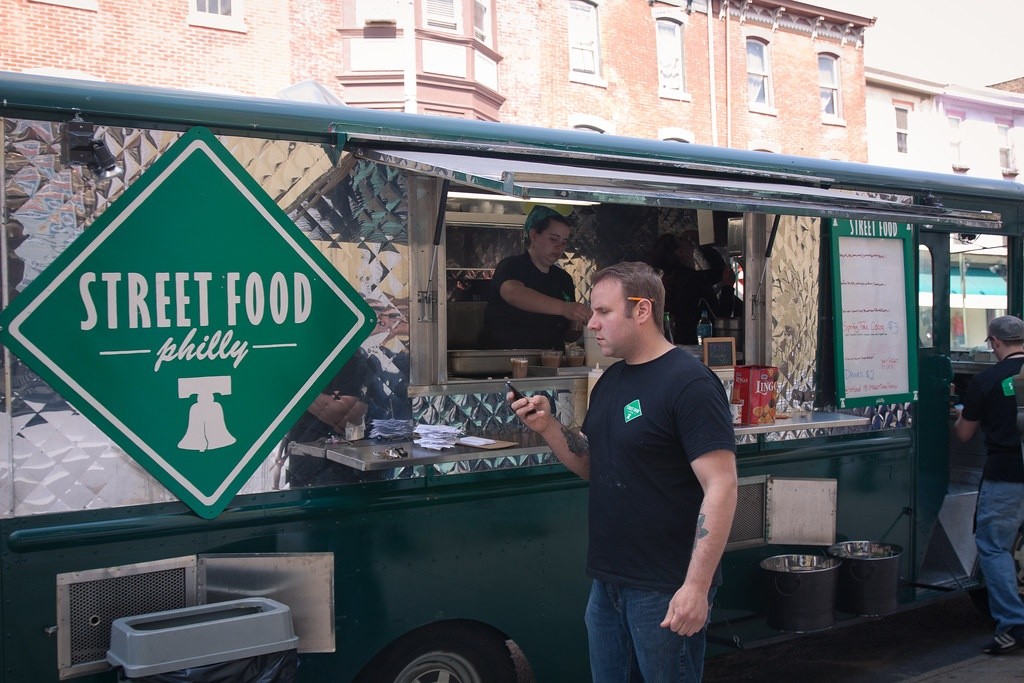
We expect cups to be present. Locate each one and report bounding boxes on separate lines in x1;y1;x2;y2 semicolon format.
510;358;528;378
540;351;564;366
566;349;585;366
568;319;583;331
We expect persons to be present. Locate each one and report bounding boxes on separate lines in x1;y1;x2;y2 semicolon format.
649;235;744;345
955;316;1024;655
475;205;592;350
286;298;409;487
505;262;739;683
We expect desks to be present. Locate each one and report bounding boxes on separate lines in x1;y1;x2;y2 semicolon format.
328;411;870;472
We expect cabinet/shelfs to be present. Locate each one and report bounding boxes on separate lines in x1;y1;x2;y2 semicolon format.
446;196;531;281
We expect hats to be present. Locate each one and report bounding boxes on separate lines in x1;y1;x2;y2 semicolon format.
984;315;1024;342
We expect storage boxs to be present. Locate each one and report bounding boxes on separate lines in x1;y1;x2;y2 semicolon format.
735;365;779;426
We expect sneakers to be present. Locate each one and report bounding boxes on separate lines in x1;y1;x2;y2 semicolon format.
980;625;1024;655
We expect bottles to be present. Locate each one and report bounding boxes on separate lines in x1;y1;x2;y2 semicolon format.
664;312;674;345
587;363;605;411
697;311;713;345
727;381;743;427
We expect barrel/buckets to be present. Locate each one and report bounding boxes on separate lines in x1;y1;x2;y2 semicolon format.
759;554;843;634
828;539;904;615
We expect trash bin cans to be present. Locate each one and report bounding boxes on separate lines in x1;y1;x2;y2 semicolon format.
97;593;307;683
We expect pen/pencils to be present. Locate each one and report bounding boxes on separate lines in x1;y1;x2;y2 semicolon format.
625;296;657;304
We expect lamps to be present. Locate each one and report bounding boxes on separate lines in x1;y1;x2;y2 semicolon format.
88;139;124;179
448;192;601;206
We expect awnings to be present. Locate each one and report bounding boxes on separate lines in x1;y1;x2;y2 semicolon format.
353;141;1002;292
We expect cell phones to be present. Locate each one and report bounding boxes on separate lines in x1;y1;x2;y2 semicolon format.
506;381;536;415
